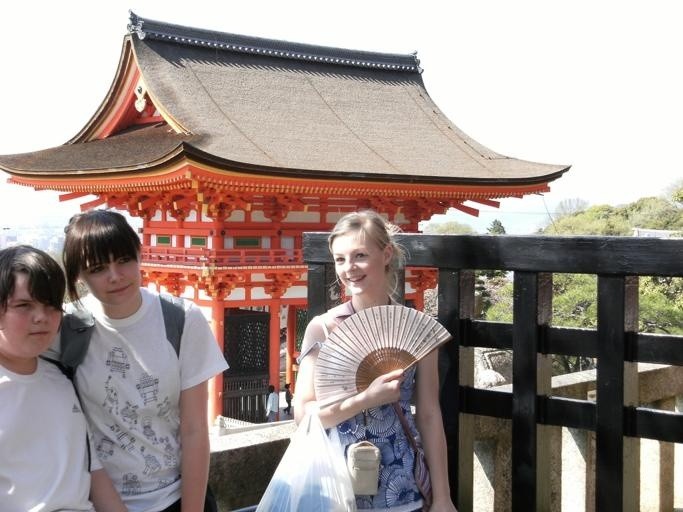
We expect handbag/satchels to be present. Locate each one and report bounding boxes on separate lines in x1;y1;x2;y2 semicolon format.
346;439;381;496
411;446;432;508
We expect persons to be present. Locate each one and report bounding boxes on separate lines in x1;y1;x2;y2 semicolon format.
0;244;127;512
283;383;293;416
264;385;279;423
294;208;458;512
46;210;232;512
